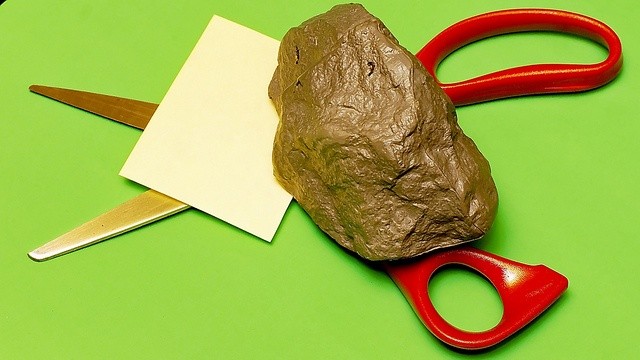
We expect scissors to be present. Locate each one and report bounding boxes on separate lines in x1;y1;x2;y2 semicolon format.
28;9;623;351
